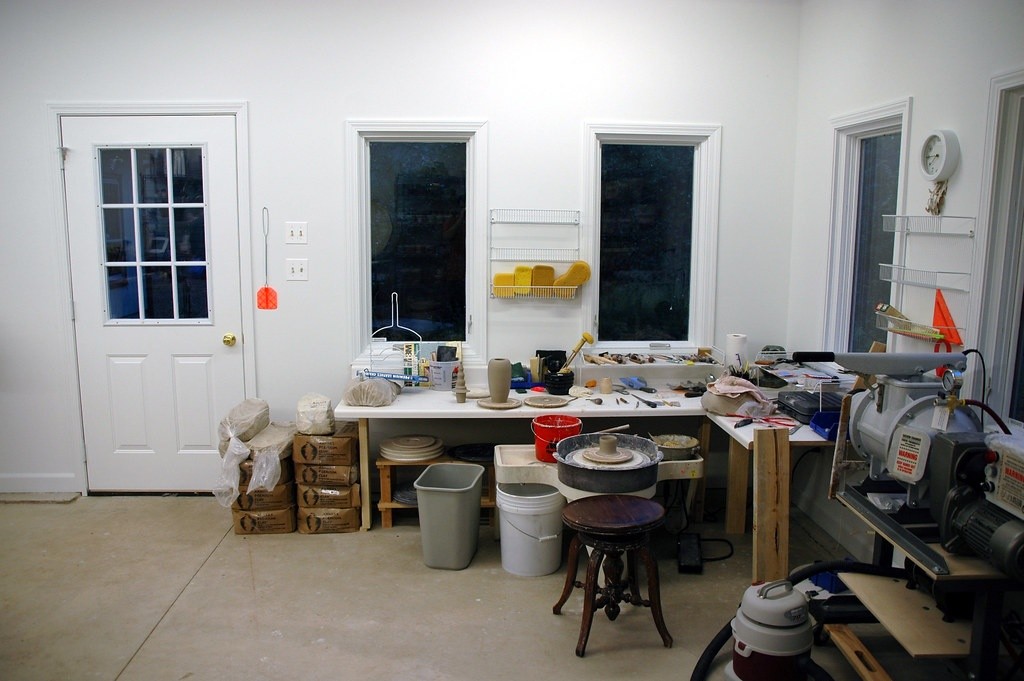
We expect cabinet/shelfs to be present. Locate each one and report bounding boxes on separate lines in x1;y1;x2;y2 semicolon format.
375;445;496;528
490;209;579;299
876;214;977;347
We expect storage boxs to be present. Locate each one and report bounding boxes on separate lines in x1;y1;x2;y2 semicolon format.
876;303;911;328
777;391;842;424
232;421;361;535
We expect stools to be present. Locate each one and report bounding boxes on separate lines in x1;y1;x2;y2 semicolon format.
552;495;674;657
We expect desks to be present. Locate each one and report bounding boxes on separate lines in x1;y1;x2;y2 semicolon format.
333;386;851;536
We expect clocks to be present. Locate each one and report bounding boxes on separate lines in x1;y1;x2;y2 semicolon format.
918;129;960;182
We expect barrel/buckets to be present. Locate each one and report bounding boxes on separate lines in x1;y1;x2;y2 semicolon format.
495;481;566;576
429;361;459;391
530;414;583;463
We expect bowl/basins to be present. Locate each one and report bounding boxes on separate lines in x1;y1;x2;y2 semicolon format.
650;433;700;461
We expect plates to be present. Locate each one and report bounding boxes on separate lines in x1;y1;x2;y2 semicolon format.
379;434;445;462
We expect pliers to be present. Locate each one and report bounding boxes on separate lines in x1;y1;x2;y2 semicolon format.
755;417;797;427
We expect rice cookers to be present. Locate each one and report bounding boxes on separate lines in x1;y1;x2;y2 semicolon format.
730;578;817;681
